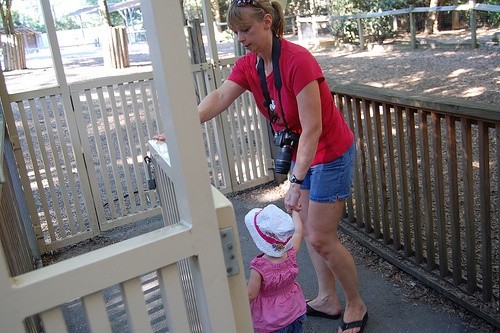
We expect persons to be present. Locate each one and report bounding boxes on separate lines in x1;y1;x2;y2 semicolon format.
153;0;368;333
244;204;307;333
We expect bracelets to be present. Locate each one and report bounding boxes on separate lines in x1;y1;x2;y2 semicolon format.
288;174;302;185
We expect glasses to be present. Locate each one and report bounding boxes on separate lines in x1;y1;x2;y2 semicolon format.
233;0;268;15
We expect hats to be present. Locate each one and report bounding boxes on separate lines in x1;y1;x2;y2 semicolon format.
242;203;298;258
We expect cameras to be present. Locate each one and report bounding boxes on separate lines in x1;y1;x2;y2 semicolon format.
273;129;300;175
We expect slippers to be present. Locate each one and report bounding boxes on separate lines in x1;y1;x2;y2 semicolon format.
337;303;368;331
305;299;342;318
